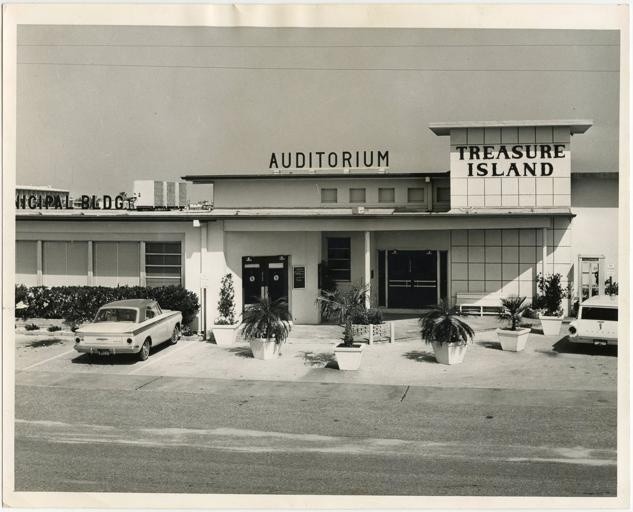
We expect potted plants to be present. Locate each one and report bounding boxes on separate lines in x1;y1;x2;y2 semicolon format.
313;276;375;371
235;294;293;359
533;272;568;335
496;296;531;350
211;273;241;348
418;294;476;364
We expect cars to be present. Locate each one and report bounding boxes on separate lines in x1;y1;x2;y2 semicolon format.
569;291;618;349
72;299;182;361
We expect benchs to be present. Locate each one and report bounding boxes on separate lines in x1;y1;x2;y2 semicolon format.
455;293;502;318
